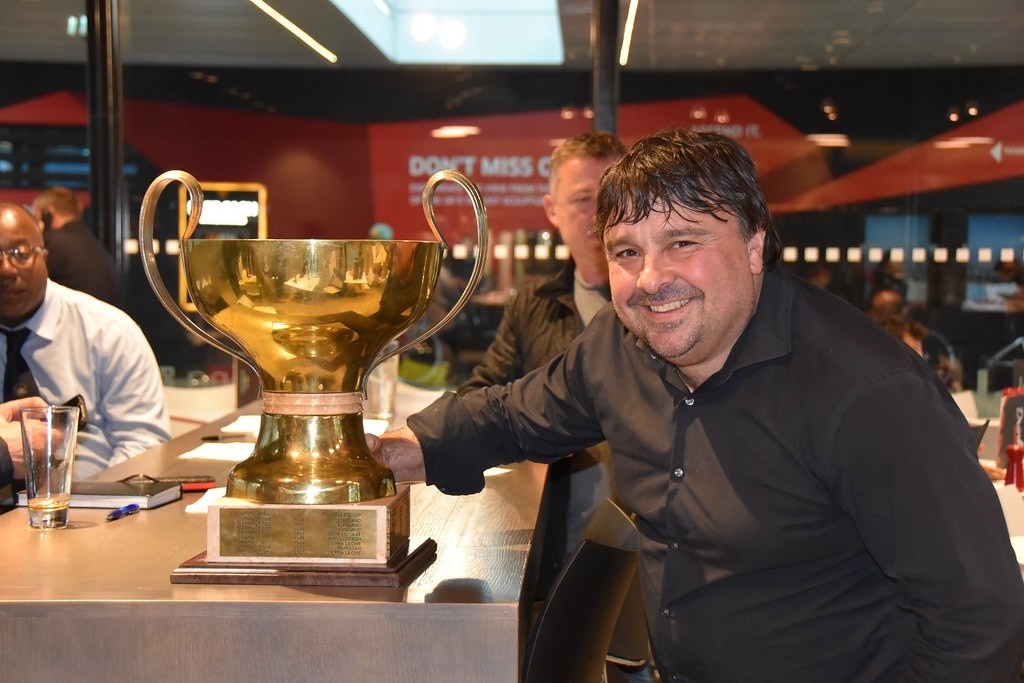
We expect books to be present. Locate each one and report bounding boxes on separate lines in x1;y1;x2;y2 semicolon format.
15;481;182;510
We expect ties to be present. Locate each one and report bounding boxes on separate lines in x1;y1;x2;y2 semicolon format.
0;327;39;403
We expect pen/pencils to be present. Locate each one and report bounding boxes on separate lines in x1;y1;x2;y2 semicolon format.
104;503;140;520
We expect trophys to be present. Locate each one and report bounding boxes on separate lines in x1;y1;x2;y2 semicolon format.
138;169;489;587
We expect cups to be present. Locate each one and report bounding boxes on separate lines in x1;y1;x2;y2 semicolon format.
363;340;399;420
20;406;80;530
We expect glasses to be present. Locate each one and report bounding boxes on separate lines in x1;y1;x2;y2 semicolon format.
875;306;909;327
0;247;45;270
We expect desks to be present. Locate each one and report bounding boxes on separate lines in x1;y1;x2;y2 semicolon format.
0;382;550;683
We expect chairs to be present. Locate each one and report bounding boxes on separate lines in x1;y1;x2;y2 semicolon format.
521;501;643;683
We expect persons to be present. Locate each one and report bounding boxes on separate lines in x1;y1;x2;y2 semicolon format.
871;291;954;385
0;396;62;489
34;187;130;317
0;199;171;483
362;131;1024;682
458;131;659;681
